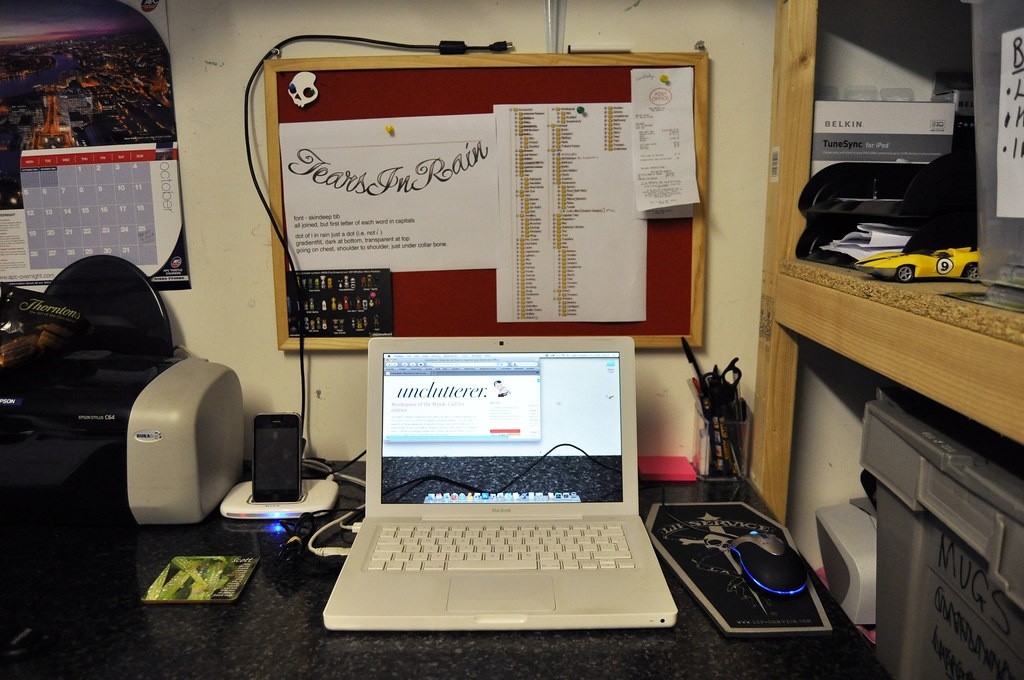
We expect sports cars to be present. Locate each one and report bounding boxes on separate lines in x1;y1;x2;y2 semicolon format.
854;246;981;285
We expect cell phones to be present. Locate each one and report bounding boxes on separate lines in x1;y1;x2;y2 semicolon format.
251;411;304;501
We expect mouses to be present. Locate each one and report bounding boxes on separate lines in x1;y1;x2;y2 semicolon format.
729;528;811;595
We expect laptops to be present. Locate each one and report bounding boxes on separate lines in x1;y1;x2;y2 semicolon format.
324;336;677;628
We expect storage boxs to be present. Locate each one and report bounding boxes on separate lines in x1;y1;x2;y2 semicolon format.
933;89;972;129
860;384;1024;680
960;0;1024;289
811;96;957;176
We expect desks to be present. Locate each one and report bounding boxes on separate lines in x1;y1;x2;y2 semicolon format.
0;458;895;680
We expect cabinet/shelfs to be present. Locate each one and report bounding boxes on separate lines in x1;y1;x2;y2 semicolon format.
797;124;978;269
754;1;1024;529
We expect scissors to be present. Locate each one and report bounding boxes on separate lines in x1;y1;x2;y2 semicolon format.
701;357;742;417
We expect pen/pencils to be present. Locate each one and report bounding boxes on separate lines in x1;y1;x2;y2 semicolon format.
680;336;749;417
